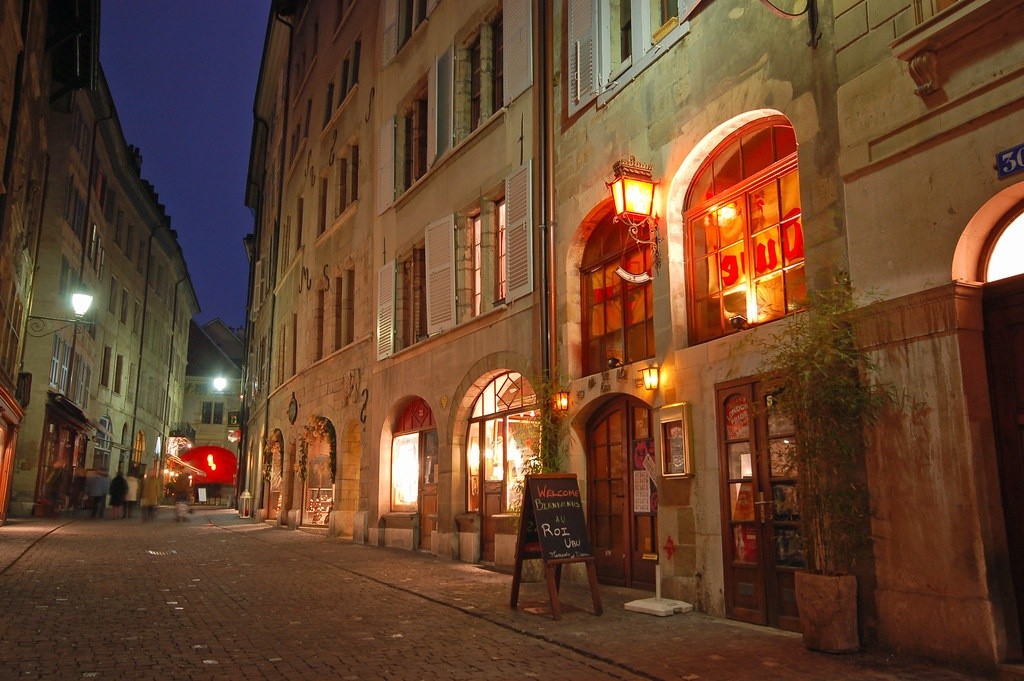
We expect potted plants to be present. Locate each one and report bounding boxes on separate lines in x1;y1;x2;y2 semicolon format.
507;360;575;583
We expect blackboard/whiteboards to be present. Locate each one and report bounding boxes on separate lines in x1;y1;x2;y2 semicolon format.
528;473;593;564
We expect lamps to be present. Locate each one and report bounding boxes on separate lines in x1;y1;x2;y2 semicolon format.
764;387;783;407
729;314;750;331
637;364;660;390
555;389;570;411
607;358;622;369
25;281;97;338
605;155;665;284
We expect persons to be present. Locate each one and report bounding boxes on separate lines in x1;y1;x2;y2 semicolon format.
141;468;162;522
122;470;139;519
88;470;110;520
108;471;128;519
